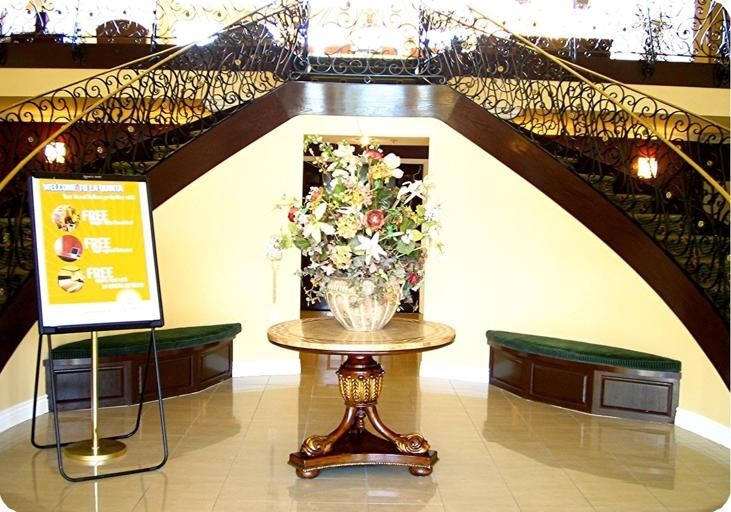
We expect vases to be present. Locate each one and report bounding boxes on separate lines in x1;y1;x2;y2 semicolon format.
318;273;406;332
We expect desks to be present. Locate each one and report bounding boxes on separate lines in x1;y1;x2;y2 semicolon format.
267;317;457;479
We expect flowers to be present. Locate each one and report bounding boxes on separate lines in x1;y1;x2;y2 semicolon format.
268;126;452;317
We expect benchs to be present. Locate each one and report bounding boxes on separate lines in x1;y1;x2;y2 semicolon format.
487;328;683;425
44;320;243;410
